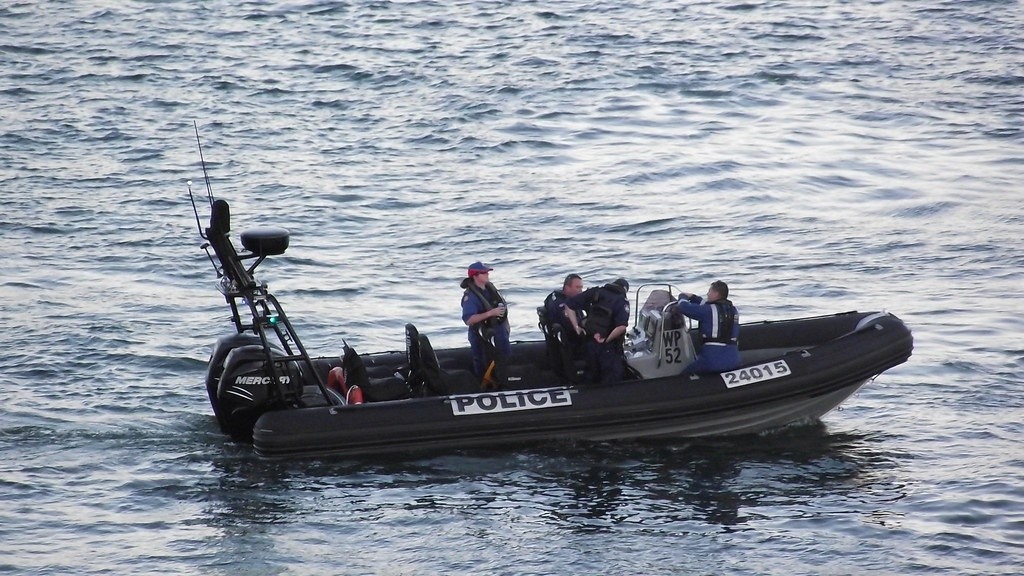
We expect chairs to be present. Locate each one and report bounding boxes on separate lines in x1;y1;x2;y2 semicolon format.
405;321;467;382
341;336;355;389
417;333;475;395
348;347;411;405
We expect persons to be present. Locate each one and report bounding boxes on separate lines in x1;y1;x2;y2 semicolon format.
458;260;513;393
534;272;585;367
675;280;742;377
564;277;632;382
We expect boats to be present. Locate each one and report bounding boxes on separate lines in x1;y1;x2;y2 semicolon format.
205;200;912;465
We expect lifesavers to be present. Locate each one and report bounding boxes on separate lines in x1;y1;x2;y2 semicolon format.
328;366;347;396
345;385;363;406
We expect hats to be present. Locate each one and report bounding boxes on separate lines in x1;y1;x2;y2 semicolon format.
468;261;494;276
